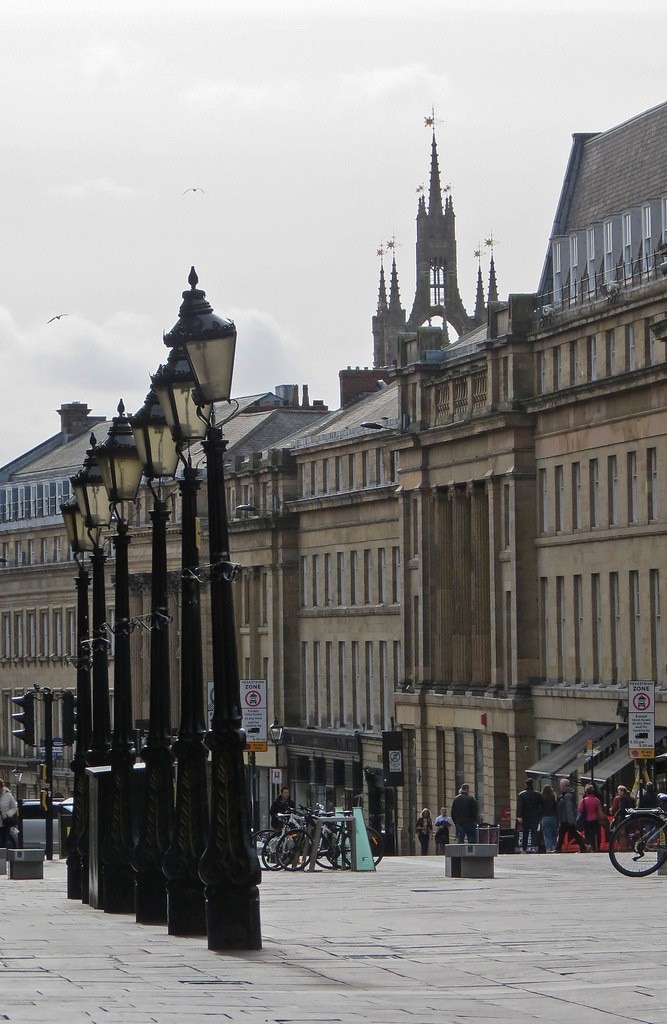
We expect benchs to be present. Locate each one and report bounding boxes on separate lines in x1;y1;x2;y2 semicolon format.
445;844;498;878
0;848;6;875
8;849;45;879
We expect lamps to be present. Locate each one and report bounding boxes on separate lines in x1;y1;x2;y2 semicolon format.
361;422;409;434
236;505;276;513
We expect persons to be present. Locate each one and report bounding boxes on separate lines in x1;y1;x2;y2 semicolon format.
270;787;294;836
451;783;478;844
517;778;658;855
435;807;453;854
0;779;17;849
415;807;433;856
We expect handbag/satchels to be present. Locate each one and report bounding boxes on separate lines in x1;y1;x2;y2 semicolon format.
2;816;19;827
575;810;587;832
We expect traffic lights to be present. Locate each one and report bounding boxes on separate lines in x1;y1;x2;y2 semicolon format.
11;692;35;745
61;690;78;746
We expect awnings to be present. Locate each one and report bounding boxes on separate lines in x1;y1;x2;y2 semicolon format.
525;723;667;788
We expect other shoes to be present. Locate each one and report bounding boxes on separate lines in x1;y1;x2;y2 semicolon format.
587;844;592;853
520;848;526;854
546;850;561;854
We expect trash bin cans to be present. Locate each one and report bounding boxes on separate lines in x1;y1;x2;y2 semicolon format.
57;797;74;859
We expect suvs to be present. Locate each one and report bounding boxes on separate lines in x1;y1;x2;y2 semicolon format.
8;799;63;853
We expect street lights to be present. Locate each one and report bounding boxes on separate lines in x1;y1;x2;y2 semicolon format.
58;492;98;900
269;716;283;796
68;431;116;906
127;388;179;924
150;345;206;935
94;399;146;913
178;266;263;951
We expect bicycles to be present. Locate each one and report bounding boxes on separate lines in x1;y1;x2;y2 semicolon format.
253;801;385;872
608;793;667;877
